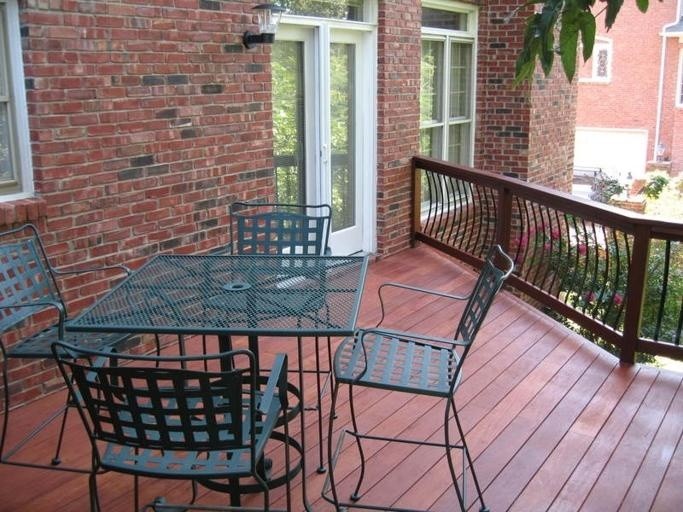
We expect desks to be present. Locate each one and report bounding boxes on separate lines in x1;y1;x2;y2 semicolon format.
70;243;370;499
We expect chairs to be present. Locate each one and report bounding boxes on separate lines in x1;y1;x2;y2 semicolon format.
47;341;295;512
316;242;513;504
1;222;124;472
193;201;340;426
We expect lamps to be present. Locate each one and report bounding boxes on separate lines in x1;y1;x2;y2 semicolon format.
245;1;285;50
